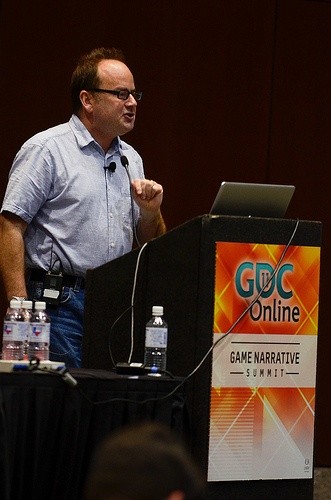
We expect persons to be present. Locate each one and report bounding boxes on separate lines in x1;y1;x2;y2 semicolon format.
86;424;207;500
0;46;169;373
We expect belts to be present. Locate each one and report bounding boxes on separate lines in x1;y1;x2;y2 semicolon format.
29;269;86;290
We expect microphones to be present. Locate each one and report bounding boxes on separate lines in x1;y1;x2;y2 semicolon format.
120;155;140;247
108;162;116;172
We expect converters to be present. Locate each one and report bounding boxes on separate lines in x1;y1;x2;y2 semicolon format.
114;362;161;375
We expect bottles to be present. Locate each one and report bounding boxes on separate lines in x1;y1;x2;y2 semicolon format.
21;299;50;361
3;300;24;361
144;305;168;377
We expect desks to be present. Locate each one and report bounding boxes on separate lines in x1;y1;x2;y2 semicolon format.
1;357;195;500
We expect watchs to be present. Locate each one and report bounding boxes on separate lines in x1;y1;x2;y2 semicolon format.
7;296;30;307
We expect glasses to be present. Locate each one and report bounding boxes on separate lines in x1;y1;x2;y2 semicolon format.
85;88;142;101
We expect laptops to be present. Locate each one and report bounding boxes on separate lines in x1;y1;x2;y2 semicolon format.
209;182;296;216
